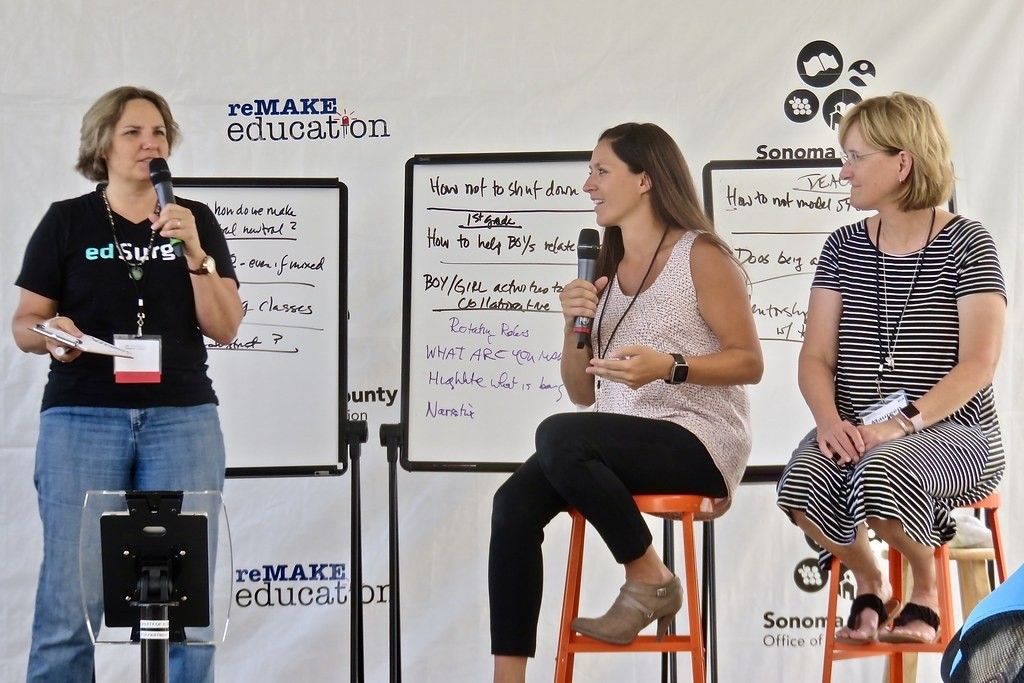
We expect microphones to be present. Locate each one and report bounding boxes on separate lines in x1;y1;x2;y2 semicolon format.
150;158;183;258
574;228;600;349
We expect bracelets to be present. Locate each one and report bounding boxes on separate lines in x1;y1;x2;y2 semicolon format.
888;414;911;437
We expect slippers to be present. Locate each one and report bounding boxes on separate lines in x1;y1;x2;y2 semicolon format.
836;593;942;645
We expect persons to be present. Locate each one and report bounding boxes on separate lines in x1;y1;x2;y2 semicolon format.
486;120;765;683
10;86;243;683
771;89;1007;647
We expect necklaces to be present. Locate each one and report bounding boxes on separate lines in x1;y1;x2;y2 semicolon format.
596;220;673;389
882;206;936;372
102;182;161;281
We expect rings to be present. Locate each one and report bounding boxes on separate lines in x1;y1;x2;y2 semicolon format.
176;220;181;228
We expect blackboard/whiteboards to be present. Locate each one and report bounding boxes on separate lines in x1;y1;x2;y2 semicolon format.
704;157;956;484
94;177;348;477
399;149;607;475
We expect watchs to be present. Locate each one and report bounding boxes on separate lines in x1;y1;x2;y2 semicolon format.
664;353;689;385
187;255;216;276
898;402;924;433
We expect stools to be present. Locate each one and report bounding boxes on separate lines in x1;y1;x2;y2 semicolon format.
554;494;715;682
821;493;1007;683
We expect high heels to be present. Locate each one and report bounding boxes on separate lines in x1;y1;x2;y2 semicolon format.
571;572;684;646
